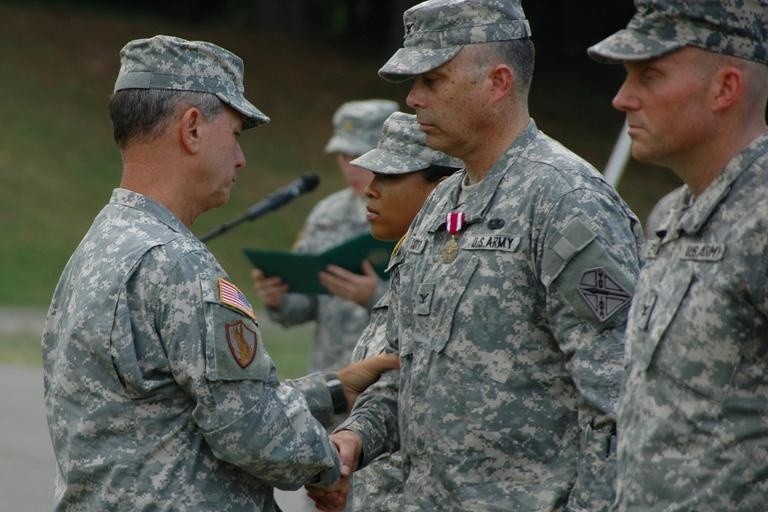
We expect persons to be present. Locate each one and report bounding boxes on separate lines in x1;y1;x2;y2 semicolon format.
39;32;400;511
336;112;459;510
293;2;642;511
572;0;766;512
250;99;404;367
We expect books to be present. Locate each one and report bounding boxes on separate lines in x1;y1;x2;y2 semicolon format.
240;230;396;297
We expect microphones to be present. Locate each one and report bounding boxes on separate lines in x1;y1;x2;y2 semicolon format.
248;172;320;224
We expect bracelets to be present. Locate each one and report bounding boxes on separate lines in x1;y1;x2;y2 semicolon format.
323;370;346;416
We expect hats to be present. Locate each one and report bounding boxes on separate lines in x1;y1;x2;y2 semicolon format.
378;1;532;83
323;98;399;157
587;0;768;65
113;33;271;132
348;111;465;175
